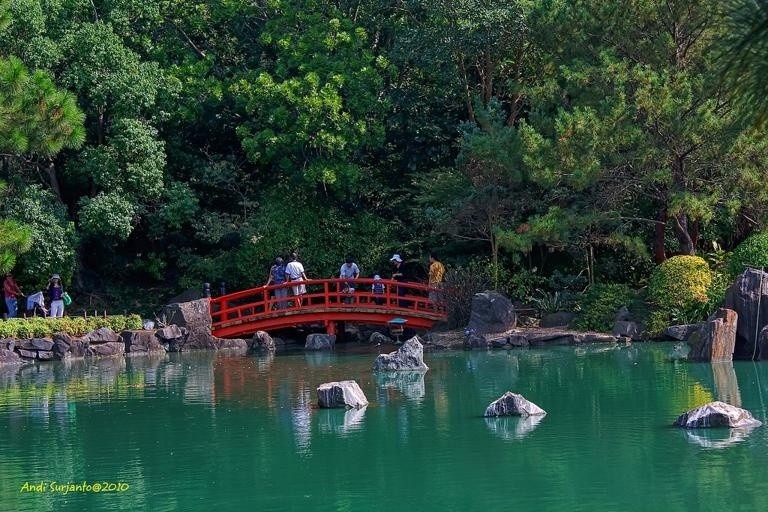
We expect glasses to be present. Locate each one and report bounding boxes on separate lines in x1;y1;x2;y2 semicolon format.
6;275;12;277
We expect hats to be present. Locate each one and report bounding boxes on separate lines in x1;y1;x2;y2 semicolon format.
390;254;403;262
374;275;380;278
51;274;61;282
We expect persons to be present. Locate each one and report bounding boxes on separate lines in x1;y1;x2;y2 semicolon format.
263;257;288;309
46;274;67;318
428;253;445;314
340;257;360;304
283;253;312;307
390;254;408;306
4;273;24;318
372;274;385;305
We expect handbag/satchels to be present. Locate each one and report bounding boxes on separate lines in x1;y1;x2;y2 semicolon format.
63;294;72;305
